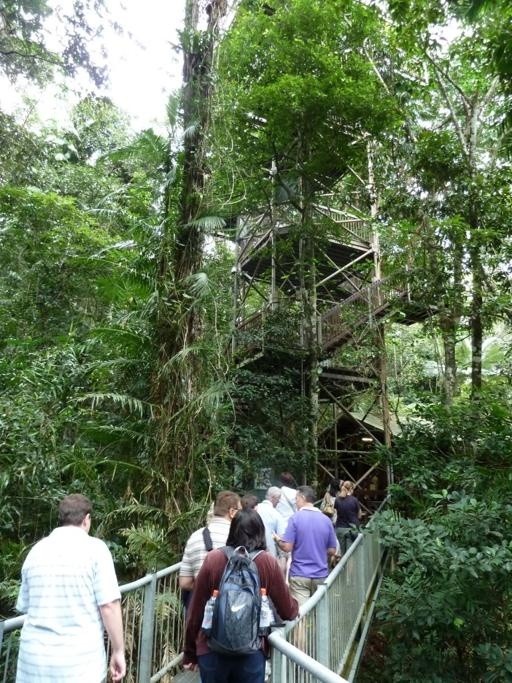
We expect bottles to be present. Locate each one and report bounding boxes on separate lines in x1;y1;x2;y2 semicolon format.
259;588;270;627
202;590;219;629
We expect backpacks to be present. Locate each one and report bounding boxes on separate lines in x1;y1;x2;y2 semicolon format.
202;547;270;656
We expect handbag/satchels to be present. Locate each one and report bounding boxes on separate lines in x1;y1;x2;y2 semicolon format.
323;504;334;517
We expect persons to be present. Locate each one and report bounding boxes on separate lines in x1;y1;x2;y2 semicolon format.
12;493;127;683
177;470;363;682
